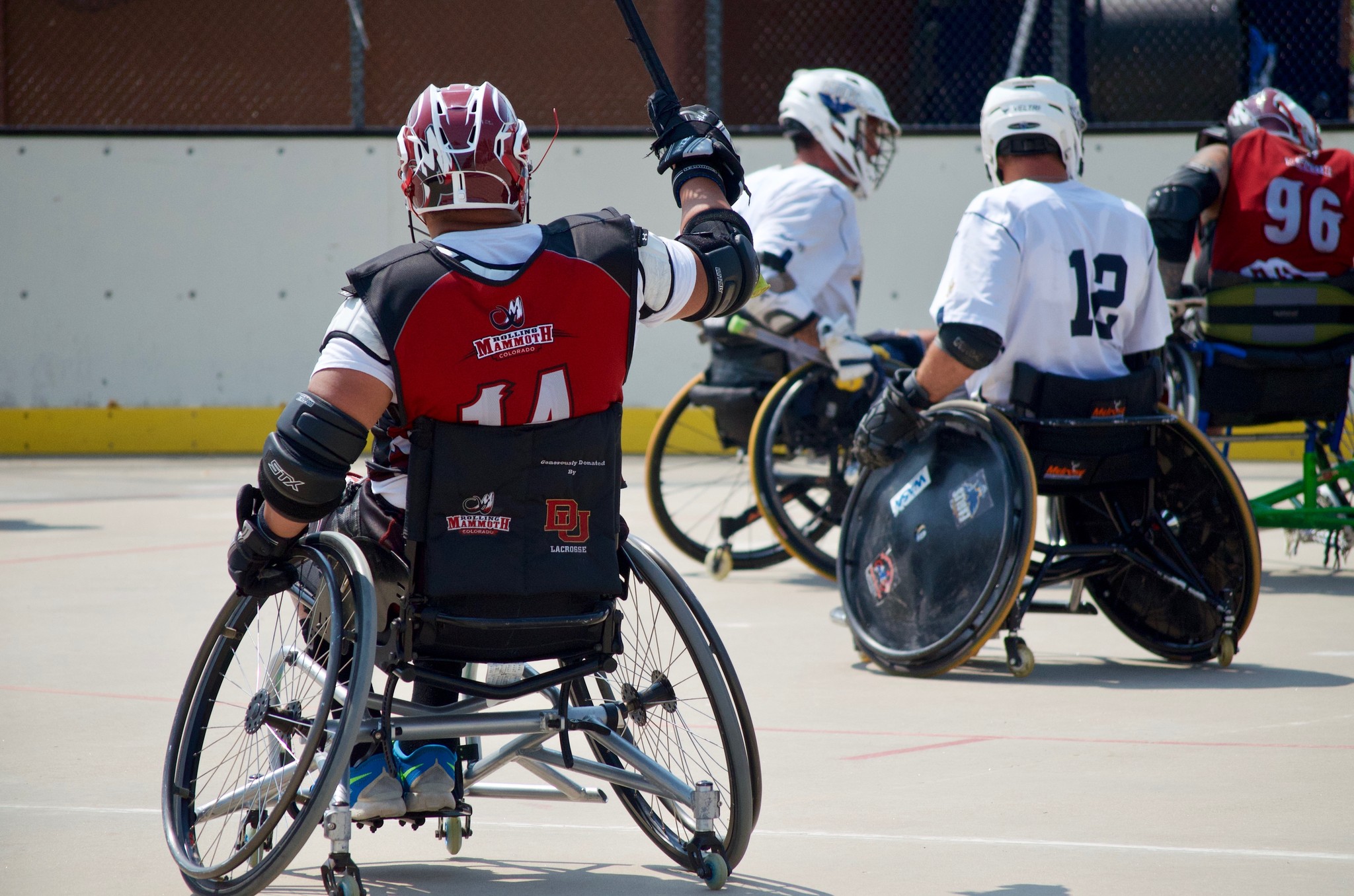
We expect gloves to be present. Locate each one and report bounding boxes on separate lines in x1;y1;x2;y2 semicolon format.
850;366;944;470
645;89;753;206
817;313;885;397
227;483;311;597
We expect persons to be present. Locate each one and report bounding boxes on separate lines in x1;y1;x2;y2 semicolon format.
850;74;1173;470
225;79;760;823
1145;87;1354;296
706;69;972;401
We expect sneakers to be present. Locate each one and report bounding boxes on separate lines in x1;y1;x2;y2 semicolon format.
308;740;407;819
392;738;457;813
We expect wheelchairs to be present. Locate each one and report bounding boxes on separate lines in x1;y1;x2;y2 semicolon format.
1156;277;1354;561
829;343;1267;672
158;394;760;896
643;307;922;584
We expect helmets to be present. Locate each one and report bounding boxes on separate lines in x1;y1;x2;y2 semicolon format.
979;74;1088;187
396;82;532;218
1226;87;1323;153
778;67;903;200
